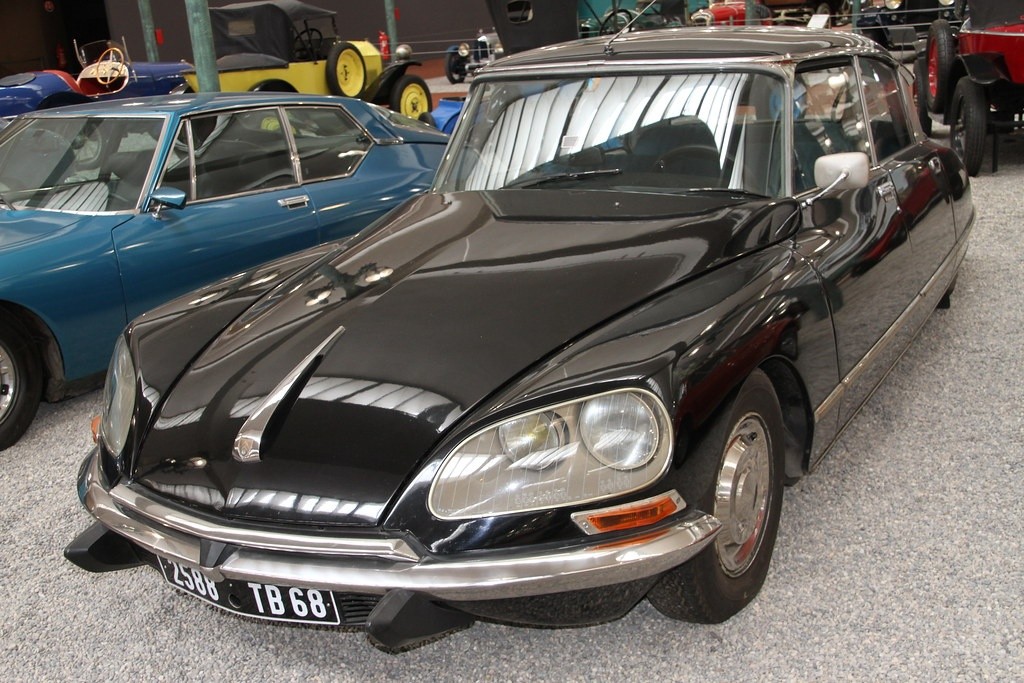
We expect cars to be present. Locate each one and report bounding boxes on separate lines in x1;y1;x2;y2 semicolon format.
167;0;432;159
856;0;969;51
63;26;974;656
445;29;504;84
914;0;1024;177
0;93;476;452
